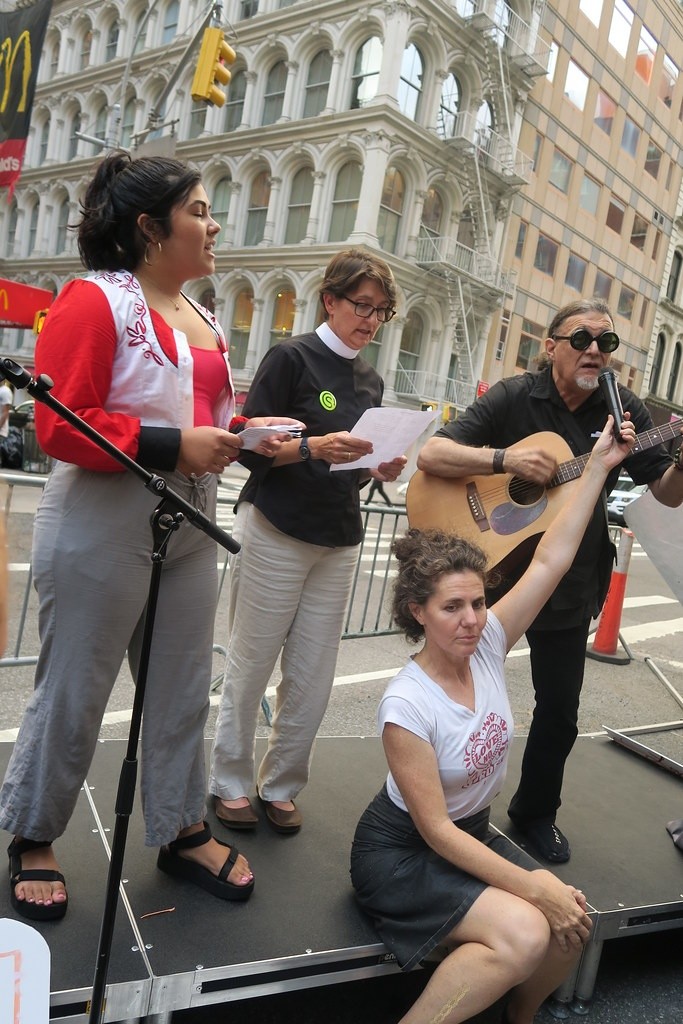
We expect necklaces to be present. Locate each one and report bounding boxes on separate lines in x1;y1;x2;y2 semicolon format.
139;273;183;310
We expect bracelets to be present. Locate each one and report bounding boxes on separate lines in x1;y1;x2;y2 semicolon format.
674;448;683;471
493;449;506;474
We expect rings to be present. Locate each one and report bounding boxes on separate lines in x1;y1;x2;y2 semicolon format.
349;453;350;458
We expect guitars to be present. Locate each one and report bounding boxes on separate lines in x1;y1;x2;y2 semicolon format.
404;417;681;584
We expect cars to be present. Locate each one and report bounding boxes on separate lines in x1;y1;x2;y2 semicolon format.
0;398;41;468
602;473;655;527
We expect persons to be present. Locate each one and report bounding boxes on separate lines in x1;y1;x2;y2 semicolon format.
417;297;683;864
208;248;408;834
350;411;636;1024
0;149;307;921
0;380;13;444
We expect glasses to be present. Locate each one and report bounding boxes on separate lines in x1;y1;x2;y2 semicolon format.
550;329;621;353
341;294;396;323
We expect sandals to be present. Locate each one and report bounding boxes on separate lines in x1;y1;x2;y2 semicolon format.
7;836;68;923
157;820;255;902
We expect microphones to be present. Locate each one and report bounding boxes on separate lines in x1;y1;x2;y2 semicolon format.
598;367;629;444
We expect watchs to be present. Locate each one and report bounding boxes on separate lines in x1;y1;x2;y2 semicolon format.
298;437;311;460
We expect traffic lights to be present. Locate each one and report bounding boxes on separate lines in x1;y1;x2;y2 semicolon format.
191;26;239;109
442;404;459;423
421;402;439;413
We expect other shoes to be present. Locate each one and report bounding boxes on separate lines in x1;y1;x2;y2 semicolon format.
211;786;259;831
514;821;571;864
257;784;303;833
